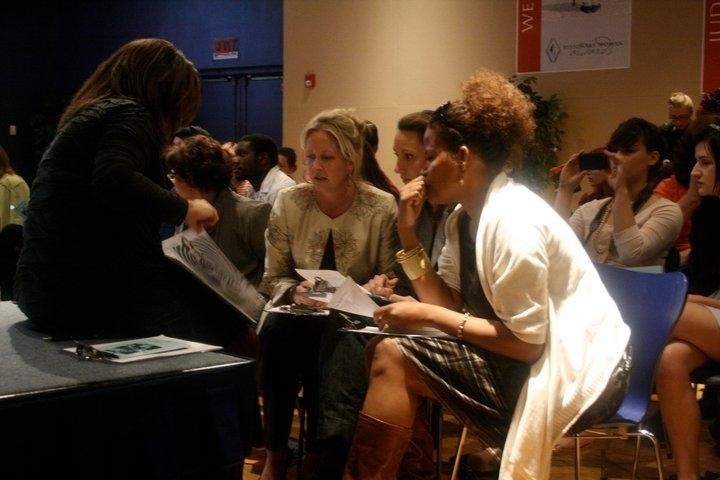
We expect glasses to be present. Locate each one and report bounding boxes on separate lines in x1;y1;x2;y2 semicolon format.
166;173;178;183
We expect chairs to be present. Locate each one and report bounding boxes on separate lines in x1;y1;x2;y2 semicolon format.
450;259;694;479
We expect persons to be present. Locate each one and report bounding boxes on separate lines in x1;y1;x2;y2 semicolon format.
1;73;719;480
17;36;264;453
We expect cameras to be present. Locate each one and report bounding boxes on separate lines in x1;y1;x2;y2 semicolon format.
579;153;609;170
